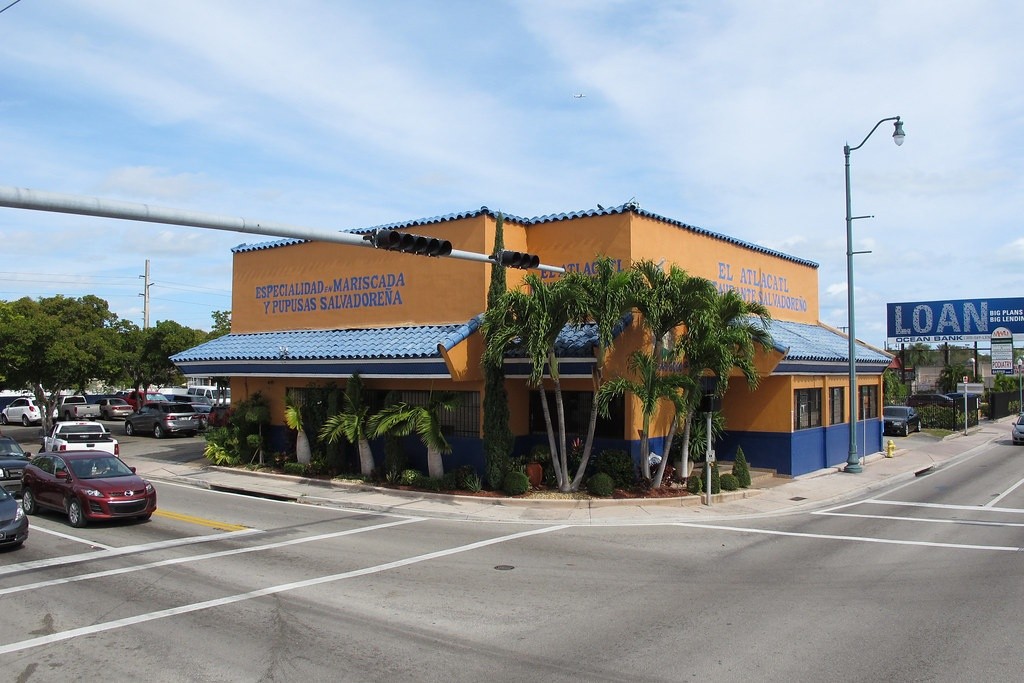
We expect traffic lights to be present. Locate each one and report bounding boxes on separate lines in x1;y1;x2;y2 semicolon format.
376;228;452;258
501;249;540;270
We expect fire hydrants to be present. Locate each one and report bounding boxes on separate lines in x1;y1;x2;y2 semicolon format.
887;440;895;458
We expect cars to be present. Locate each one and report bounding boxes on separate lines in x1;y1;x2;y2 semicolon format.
883;404;922;437
124;401;199;439
115;384;231;422
94;397;134;421
1011;412;1024;446
1;396;59;427
0;483;29;552
21;449;157;529
0;434;34;500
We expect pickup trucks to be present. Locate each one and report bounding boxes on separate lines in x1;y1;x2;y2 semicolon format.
39;420;119;459
56;395;101;421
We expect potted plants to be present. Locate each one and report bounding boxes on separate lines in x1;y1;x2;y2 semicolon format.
672;410;726;478
518;450;549;487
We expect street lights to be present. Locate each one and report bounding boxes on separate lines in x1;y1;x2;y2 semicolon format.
963;374;969;435
1017;358;1023;414
843;115;907;475
